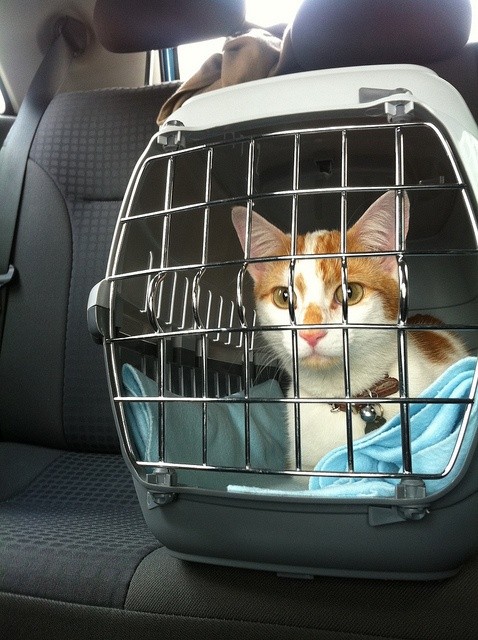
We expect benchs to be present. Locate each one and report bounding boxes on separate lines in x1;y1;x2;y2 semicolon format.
1;0;477;640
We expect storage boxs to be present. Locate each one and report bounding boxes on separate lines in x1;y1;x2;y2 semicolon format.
87;63;478;575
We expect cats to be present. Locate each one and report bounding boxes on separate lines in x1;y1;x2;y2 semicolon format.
231;187;468;484
